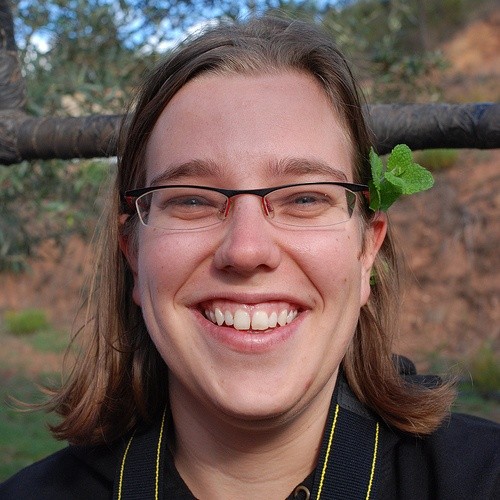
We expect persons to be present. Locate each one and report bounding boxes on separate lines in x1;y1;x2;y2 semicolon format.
1;10;500;500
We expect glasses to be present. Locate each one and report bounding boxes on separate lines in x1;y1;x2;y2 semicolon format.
123;182;372;229
